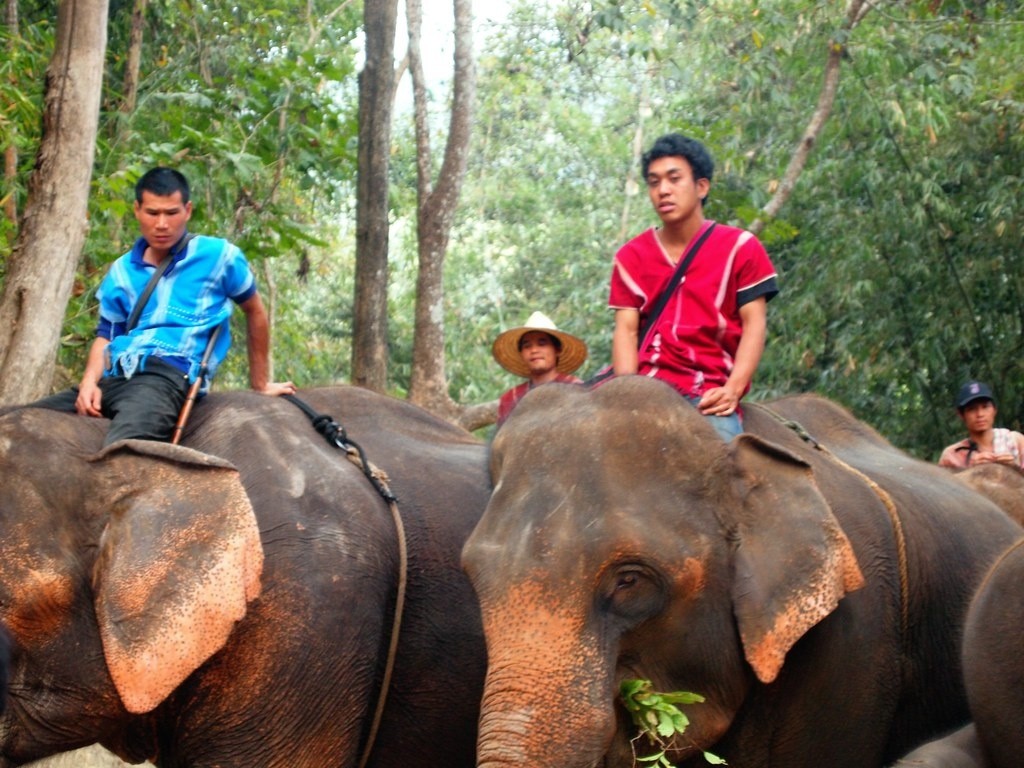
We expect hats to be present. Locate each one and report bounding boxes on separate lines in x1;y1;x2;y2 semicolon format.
493;310;588;377
956;380;995;407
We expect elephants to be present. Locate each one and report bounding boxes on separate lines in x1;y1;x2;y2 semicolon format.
456;379;1024;768
2;382;491;768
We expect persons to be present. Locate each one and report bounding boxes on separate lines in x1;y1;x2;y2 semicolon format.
22;166;297;454
588;132;781;446
493;330;587;429
938;379;1024;473
